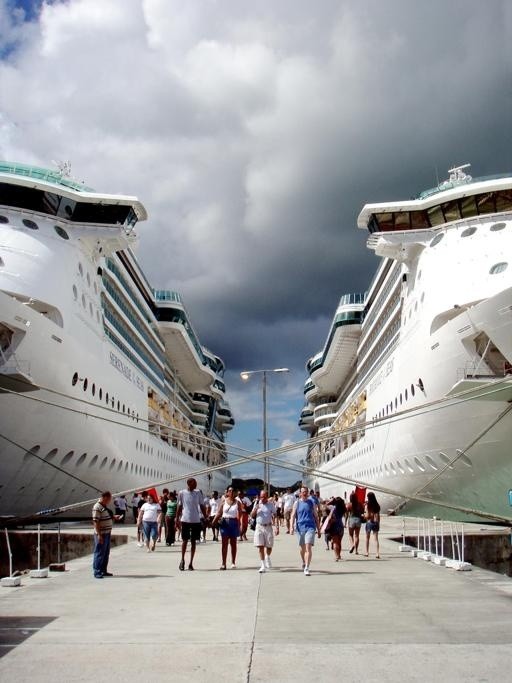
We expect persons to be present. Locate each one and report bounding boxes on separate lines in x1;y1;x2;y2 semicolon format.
92;490;120;578
114;479;380;575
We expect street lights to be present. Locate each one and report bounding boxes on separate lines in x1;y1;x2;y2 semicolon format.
237;363;291;502
251;436;281;499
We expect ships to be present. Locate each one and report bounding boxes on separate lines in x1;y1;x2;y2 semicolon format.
0;155;241;522
295;161;512;539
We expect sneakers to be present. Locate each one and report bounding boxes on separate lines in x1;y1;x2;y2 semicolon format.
178;559;185;570
259;555;271;572
94;571;113;578
302;560;311;574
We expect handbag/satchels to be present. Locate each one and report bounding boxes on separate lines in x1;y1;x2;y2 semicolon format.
219;517;227;528
249;519;255;530
